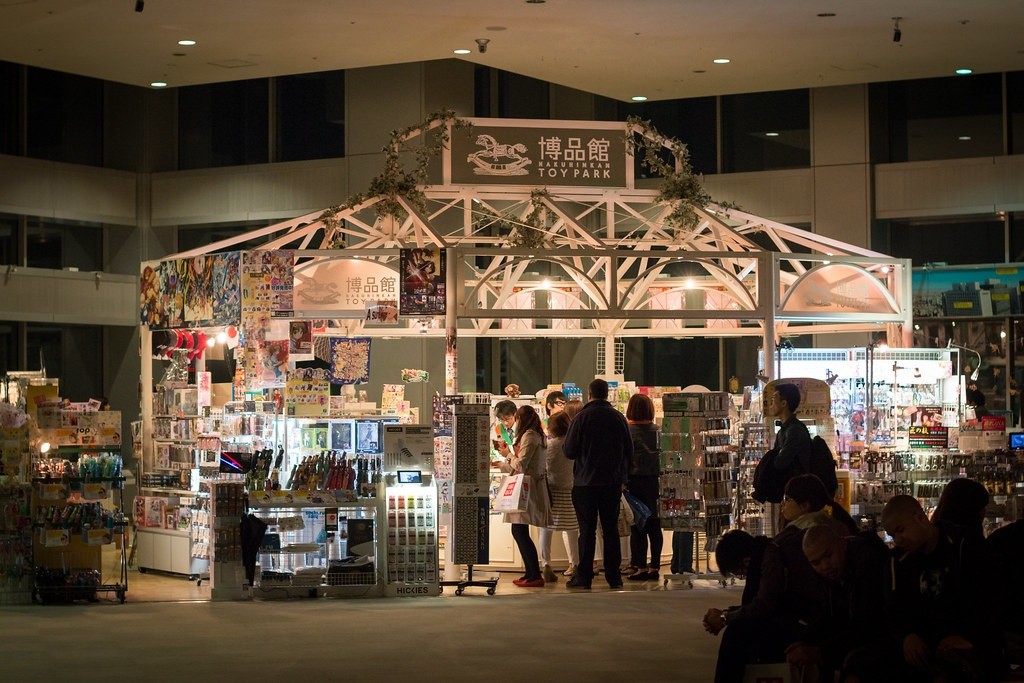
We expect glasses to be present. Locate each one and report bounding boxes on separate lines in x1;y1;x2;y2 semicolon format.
783;494;793;503
737;574;743;580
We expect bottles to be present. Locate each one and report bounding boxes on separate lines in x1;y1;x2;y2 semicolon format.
1;451;125;607
565;384;635;419
170;435;382;593
496;435;506;450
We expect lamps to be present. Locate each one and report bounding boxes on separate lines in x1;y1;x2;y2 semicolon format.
913;330;981;380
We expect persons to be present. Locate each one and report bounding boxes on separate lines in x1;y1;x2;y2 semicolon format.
768;384;812;532
562;379;635;589
671;532;704;583
967;390;994;421
703;472;1024;683
490;392;663;586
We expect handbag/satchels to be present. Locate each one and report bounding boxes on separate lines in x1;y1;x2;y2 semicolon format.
491;469;531;514
597;489;653;538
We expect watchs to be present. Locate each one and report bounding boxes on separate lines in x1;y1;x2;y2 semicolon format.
720;611;728;627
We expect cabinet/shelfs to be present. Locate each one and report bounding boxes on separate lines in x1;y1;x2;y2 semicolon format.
28;474;128;602
247;495;388;599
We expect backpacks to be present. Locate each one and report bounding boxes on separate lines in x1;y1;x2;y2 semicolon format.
793;421;839;491
640;431;662;476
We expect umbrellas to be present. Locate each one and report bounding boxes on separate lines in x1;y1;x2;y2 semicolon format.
241;498;268;588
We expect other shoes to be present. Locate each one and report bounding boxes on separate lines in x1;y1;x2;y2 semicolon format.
627;572;649;580
567;574;592;589
610;579;624;590
621;566;638;575
648;571;660;580
544;565;558;583
561;568;574;576
592;566;599;575
513;574;544;587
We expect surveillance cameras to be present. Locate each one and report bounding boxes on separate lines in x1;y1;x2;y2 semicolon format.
475;39;489;53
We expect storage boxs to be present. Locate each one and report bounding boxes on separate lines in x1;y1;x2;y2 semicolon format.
133;496;179;527
